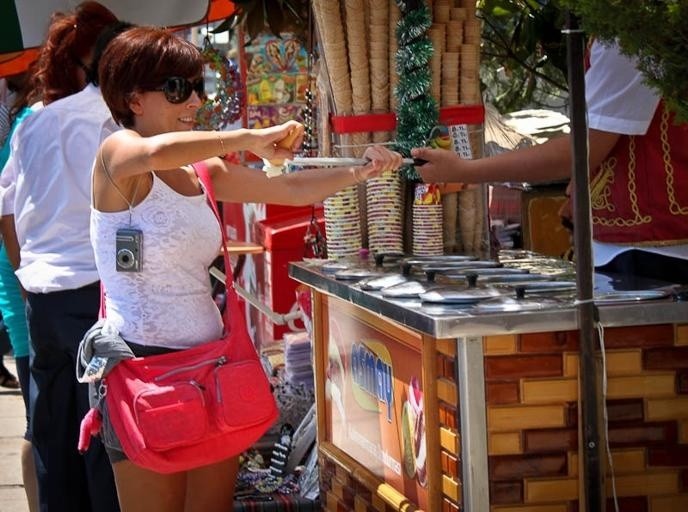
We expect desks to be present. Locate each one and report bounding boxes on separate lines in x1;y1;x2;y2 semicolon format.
200;242;264;317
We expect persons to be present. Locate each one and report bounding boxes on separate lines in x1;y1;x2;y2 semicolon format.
88;24;404;510
408;29;688;299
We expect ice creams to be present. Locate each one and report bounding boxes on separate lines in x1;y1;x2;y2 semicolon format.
262;124;304;179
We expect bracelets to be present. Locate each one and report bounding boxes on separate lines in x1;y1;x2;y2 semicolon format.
208;128;228;158
350;163;369;188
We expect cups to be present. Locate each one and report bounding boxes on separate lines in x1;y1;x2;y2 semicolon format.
365;165;404;256
412;180;445;259
320;164;362;259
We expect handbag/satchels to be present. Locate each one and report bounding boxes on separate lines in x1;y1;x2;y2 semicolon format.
101;288;279;475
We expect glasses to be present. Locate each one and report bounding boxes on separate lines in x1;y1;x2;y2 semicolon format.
146;77;204;103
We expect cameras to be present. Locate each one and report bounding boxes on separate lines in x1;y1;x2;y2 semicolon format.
114;228;145;273
